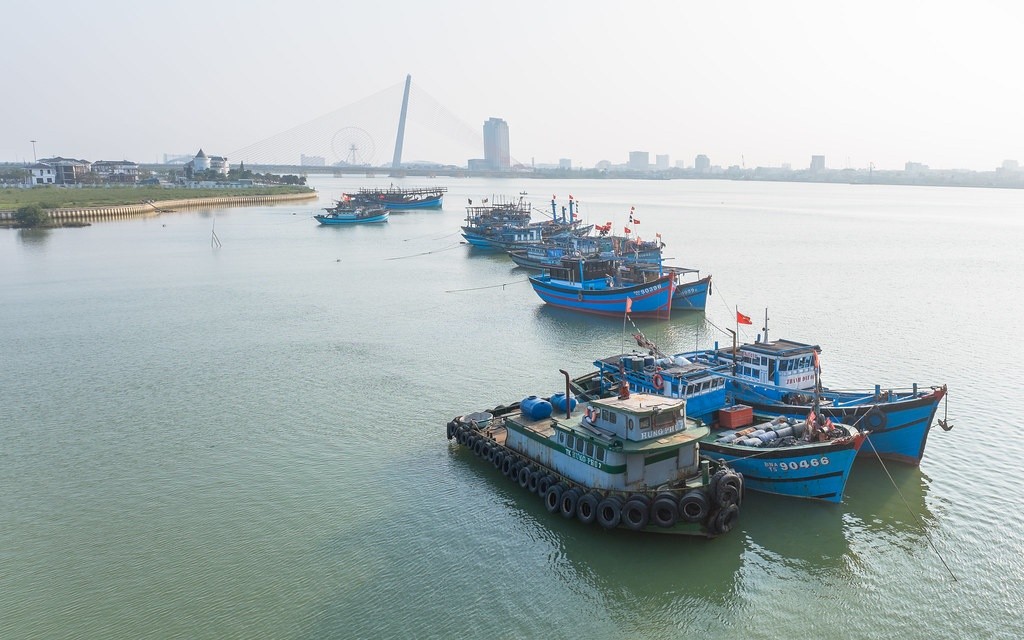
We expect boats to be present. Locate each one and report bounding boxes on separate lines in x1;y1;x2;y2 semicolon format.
359;184;447;209
446;307;953;538
314;194;390;224
460;193;712;320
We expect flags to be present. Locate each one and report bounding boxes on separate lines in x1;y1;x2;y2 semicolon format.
814;351;819;369
634;219;640;224
656;233;661;237
737;311;752;324
637;237;641;245
572;213;577;217
595;222;611;230
624;227;631;233
569;195;573;199
553;194;555;199
627;297;632;312
468;199;472;204
380;194;384;199
482;198;488;203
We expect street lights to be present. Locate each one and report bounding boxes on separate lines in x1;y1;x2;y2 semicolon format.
30;141;36;164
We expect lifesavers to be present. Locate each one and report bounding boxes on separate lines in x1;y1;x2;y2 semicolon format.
653;374;663;390
863;409;886;431
578;294;582;301
506;243;511;247
446;421;745;535
842;416;861;434
574;250;581;257
585;405;596;423
